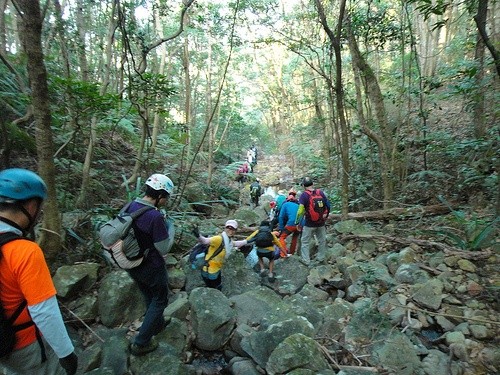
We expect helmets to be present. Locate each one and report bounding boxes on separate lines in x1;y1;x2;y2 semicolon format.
0;168;48;203
145;174;174;196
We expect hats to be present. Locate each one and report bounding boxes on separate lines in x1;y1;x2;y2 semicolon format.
225;220;238;230
269;201;276;208
256;178;260;181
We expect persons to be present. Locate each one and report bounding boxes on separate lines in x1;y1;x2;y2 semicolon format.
254;220;290;279
0;167;79;375
192;219;248;291
117;173;176;353
250;178;261;206
276;190;302;257
290;176;331;264
236;145;258;181
268;193;287;237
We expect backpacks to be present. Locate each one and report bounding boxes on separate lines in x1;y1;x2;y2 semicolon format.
97;201;154;271
304;189;325;222
251;187;259;198
256;231;274;248
188;234;224;270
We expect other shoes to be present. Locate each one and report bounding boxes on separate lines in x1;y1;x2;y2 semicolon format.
260;268;266;274
153;318;171;335
268;273;276;282
133;340;158;356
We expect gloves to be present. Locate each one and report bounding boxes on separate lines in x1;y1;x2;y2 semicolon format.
247;238;257;244
59;352;79;375
192;230;199;238
